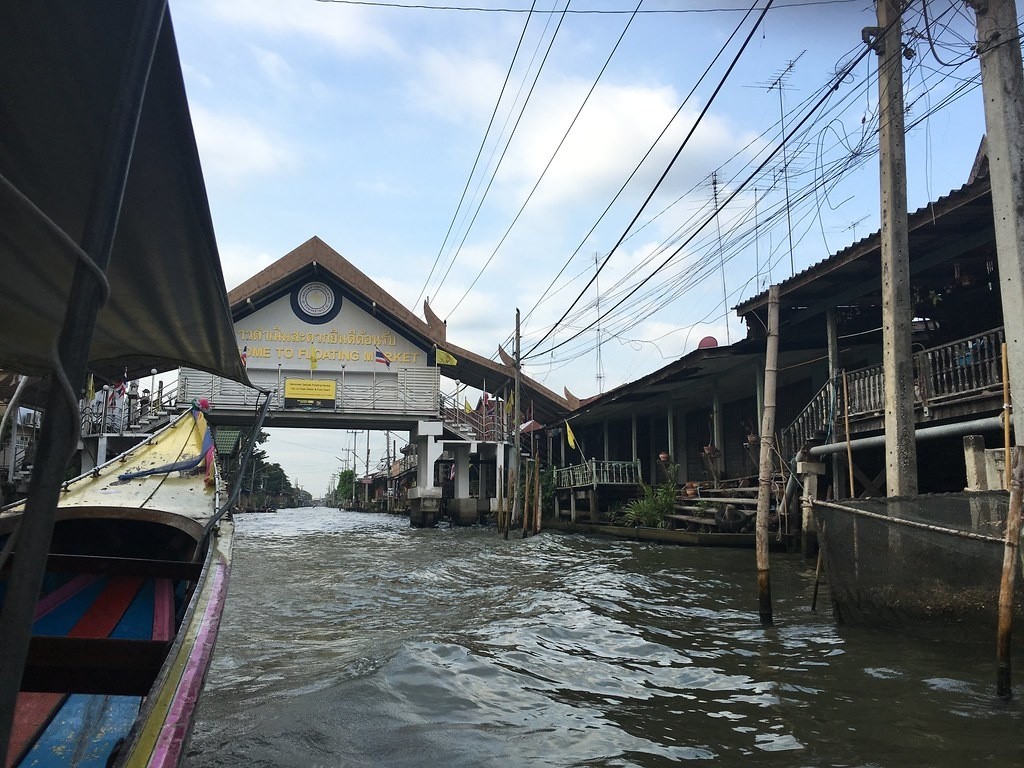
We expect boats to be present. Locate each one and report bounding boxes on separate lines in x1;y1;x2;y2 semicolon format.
0;0;275;767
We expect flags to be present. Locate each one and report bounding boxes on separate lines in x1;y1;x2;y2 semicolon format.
484;386;488;405
241;347;247;366
87;375;126;411
376;347;391;367
466;401;472;413
311;346;317;369
450;462;455;480
567;424;575;449
437;349;457;366
506;393;513;413
